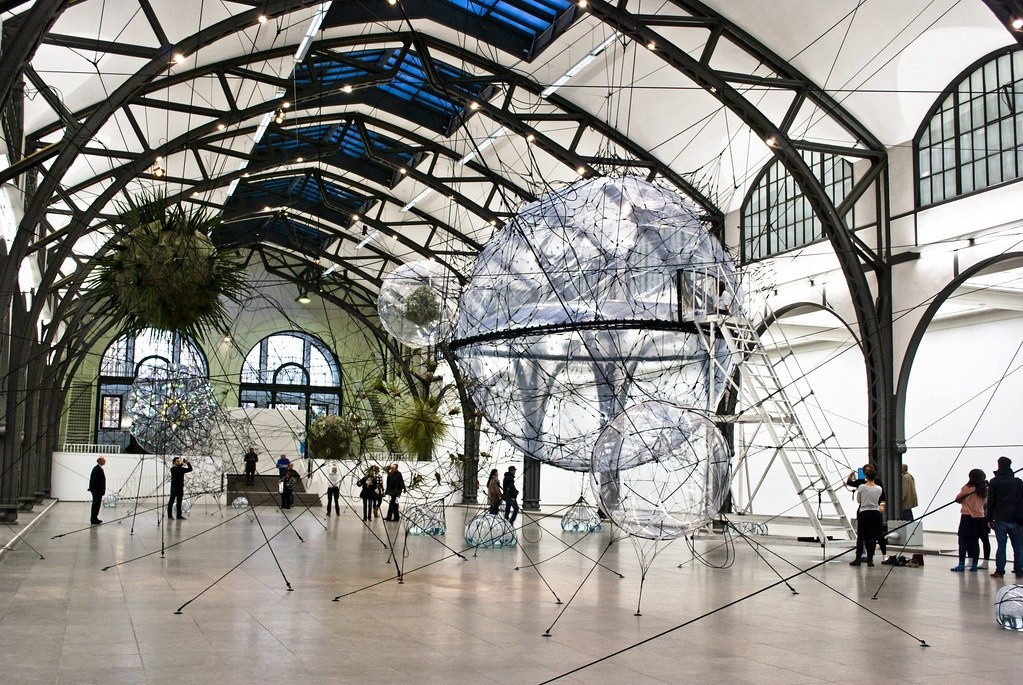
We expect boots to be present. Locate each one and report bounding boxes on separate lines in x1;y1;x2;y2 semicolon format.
965;558;974;567
969;564;978;572
977;560;988;569
950;562;965;571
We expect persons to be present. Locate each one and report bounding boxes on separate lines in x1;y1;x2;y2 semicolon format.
951;469;991;571
167;457;193;520
276;454;290;480
326;466;341;516
383;464;406;521
279;463;300;509
847;463;888;567
988;457;1023;577
486;469;502;515
357;466;384;522
901;464;918;520
503;466;519;525
87;457;107;524
244;448;258;486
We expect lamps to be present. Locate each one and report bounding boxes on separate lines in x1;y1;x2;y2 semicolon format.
298;288;311;305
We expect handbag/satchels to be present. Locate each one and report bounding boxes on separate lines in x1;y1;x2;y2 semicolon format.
278;482;283;493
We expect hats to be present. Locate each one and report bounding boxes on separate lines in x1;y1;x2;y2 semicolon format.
508;465;517;471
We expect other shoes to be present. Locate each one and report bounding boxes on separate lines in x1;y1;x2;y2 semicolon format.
91;519;102;524
868;561;875;566
362;519;367;521
177;516;185;520
383;518;391;521
881;554;925;567
373;510;379;517
1012;570;1015;573
990;572;1004;579
850;560;859;566
367;517;372;521
391;519;399;522
168;517;174;520
